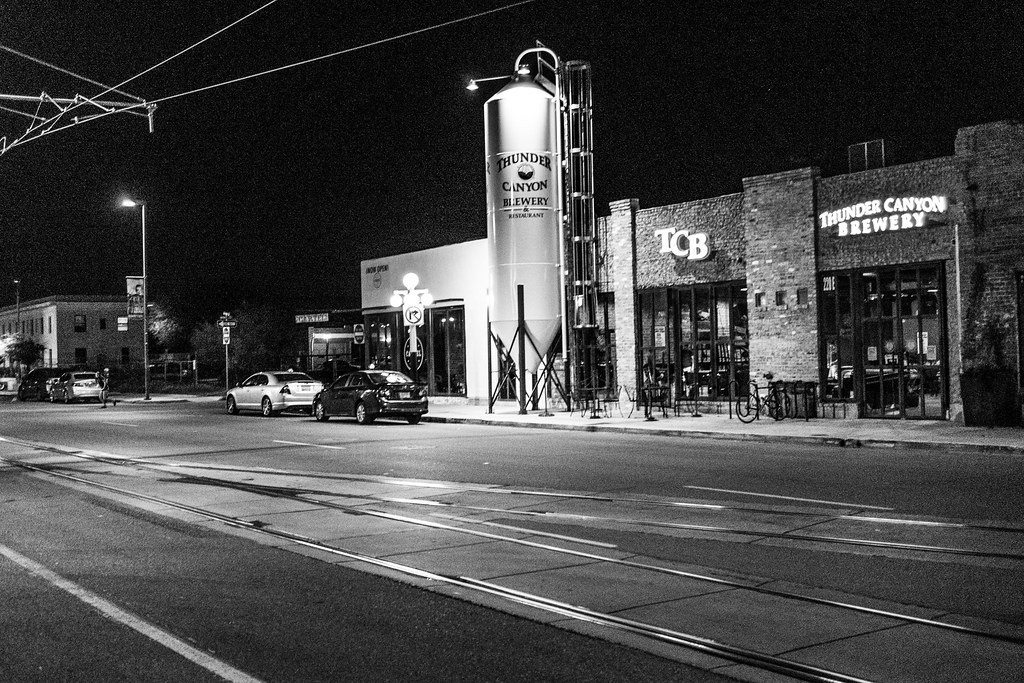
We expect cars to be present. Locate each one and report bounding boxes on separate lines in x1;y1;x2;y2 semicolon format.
17;366;68;400
48;369;102;402
225;368;325;415
311;365;429;425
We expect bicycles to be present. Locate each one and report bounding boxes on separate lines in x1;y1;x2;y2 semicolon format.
736;371;791;423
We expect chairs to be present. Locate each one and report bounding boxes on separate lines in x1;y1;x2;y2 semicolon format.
568;377;672;421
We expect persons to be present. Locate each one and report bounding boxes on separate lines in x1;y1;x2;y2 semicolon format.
99;368;117;409
20;377;28;405
95;372;108;391
